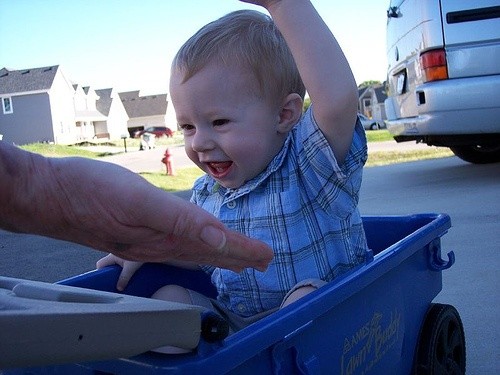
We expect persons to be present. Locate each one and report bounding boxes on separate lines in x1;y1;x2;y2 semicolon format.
95;0;369;353
0;139;275;274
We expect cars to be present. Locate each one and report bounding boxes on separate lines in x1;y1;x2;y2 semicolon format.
358;113;386;130
139;126;173;139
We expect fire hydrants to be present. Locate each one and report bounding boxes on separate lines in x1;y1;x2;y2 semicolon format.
161;148;174;176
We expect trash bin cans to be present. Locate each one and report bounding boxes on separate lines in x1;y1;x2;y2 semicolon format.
143;133;156;149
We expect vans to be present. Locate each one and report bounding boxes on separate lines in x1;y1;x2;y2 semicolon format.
384;0;500;166
